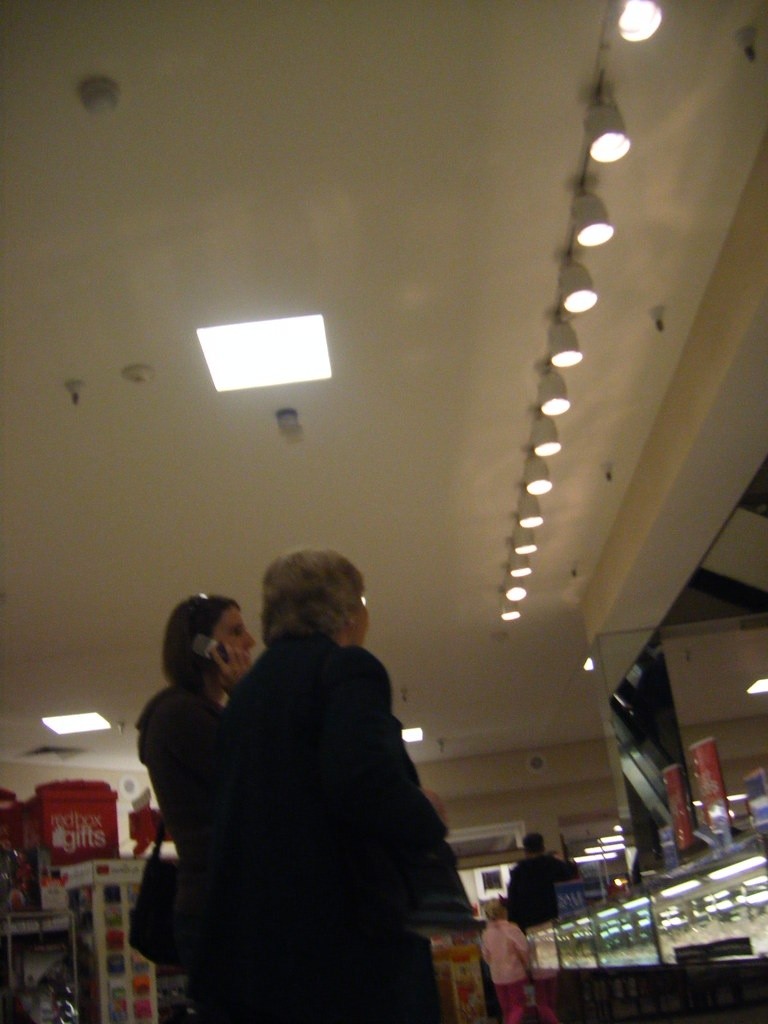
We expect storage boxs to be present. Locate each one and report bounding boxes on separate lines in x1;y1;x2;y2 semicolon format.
0;787;23;852
19;779;121;866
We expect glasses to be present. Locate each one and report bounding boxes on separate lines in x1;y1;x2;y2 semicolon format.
187;591;207;640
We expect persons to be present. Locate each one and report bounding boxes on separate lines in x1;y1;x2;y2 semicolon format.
139;596;251;1024
507;833;578;935
482;898;528;1024
532;968;560;1024
217;550;457;1024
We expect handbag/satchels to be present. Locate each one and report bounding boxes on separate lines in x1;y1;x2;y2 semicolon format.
128;854;188;970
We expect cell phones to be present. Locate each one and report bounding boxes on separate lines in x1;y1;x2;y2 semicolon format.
192;634;229;664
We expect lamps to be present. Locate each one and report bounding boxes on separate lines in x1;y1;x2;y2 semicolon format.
548;320;583;368
518;492;544;528
524;454;552;496
530;415;561;457
500;593;522;621
510;550;533;577
558;258;598;313
505;573;527;601
512;523;537;555
582;104;631;163
538;370;571;417
611;0;662;43
570;192;615;248
